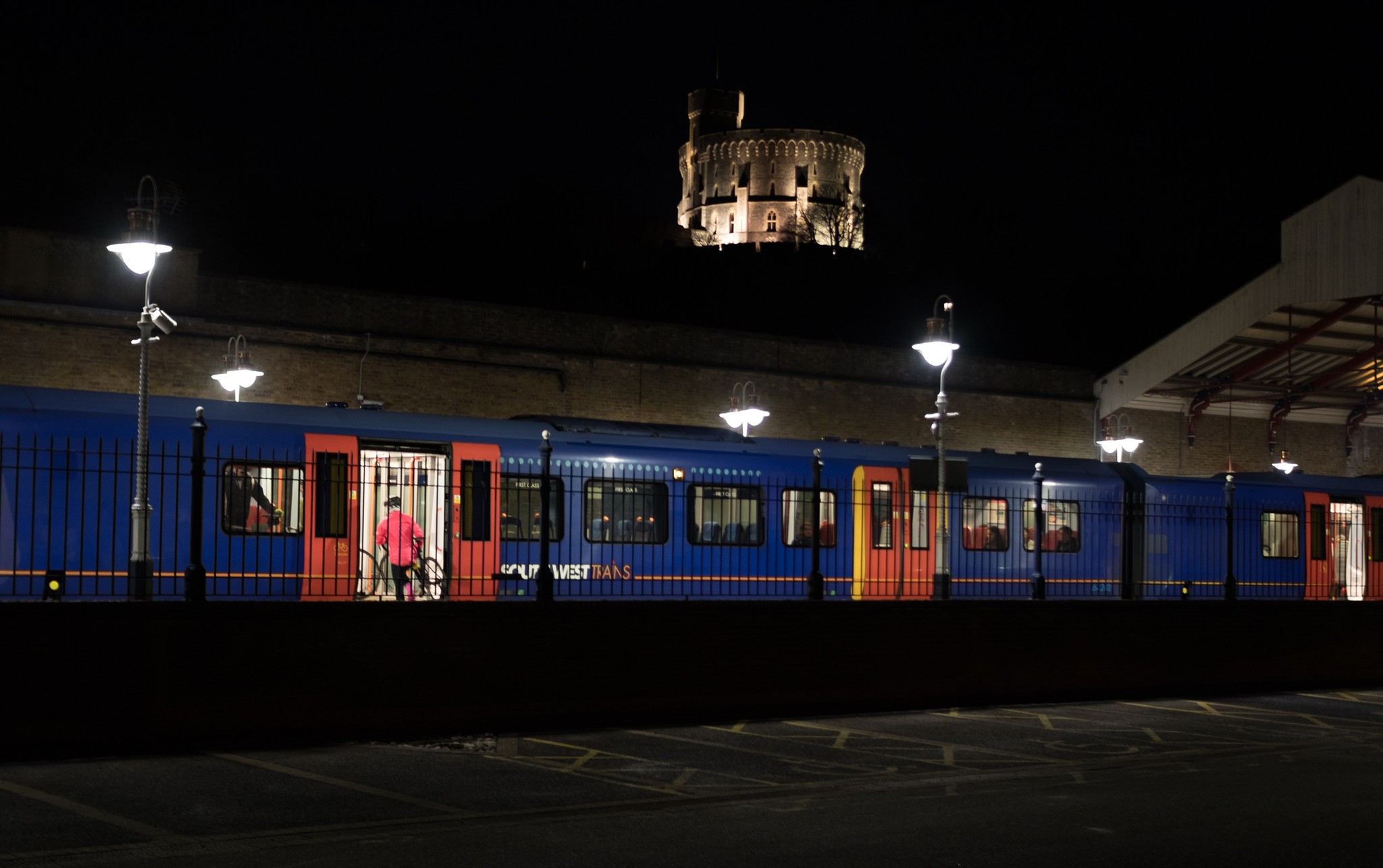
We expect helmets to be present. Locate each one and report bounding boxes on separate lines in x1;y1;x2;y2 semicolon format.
383;495;401;506
234;465;253;472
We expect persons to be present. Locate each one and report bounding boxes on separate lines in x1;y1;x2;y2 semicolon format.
1055;526;1076;551
790;522;825;545
375;496;424;601
1330;534;1350;597
983;525;1007;551
222;464;285;532
1023;527;1034;550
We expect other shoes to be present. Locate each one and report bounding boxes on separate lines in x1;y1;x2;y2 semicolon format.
404;582;416;601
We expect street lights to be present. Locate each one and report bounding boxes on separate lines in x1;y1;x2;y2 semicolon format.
209;335;261;402
717;382;771;437
1092;413;1142;465
911;296;949;601
108;175;173;596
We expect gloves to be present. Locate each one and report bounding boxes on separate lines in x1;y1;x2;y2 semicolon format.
274;508;284;518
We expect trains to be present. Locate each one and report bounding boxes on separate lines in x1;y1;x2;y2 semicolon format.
0;382;1383;605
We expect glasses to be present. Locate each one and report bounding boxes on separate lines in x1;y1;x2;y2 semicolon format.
238;470;245;474
